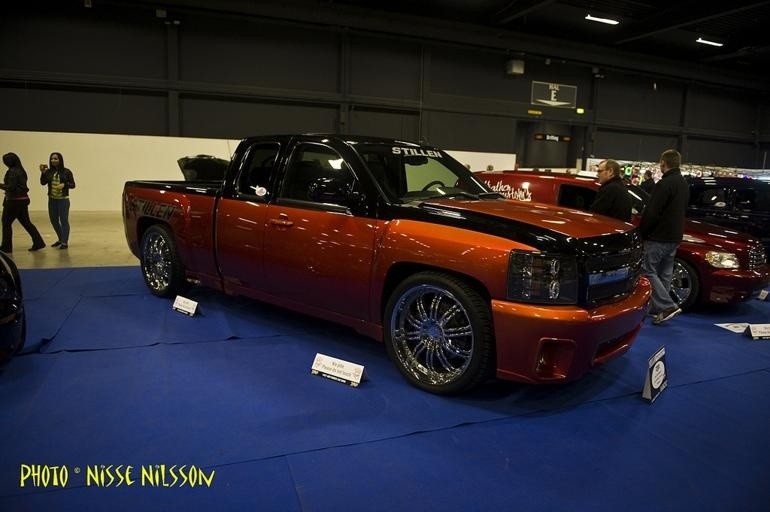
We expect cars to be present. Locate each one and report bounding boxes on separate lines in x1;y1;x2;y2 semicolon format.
0;251;27;359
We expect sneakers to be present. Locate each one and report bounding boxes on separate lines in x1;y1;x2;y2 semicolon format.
1;243;12;254
28;242;47;252
647;305;682;326
51;241;69;251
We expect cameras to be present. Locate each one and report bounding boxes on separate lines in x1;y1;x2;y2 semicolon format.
42;164;48;168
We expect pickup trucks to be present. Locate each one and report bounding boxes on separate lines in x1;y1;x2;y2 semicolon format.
121;133;652;394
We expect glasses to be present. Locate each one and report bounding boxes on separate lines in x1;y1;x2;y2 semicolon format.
596;169;607;174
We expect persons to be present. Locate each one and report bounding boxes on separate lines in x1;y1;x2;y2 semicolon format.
589;160;632;222
40;153;75;249
640;150;689;324
0;153;45;253
620;165;655;193
